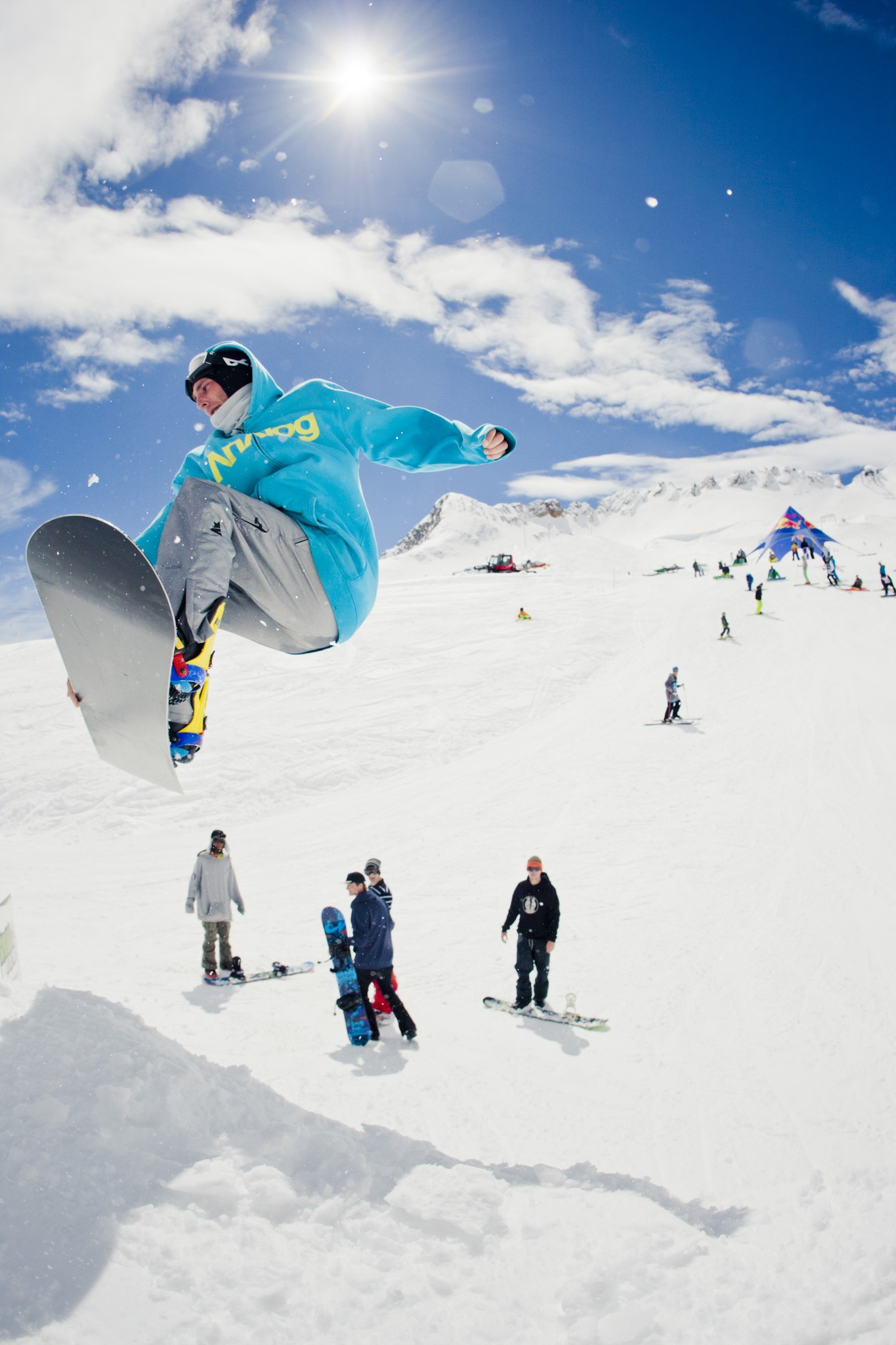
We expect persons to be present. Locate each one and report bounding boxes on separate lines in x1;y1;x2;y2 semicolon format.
664;666;684;724
518;609;529;622
364;860;394;1011
702;537;894;643
184;826;250;983
65;335;523;770
342;873;419;1046
501;855;562;1011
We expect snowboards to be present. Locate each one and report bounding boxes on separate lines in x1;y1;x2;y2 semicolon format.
204;961;314;986
482;995;609;1035
28;512;201;792
320;907;372;1046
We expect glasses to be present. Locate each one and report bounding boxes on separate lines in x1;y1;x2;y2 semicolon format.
364;867;378;875
528;866;541;871
212;838;225;844
184;351;214;398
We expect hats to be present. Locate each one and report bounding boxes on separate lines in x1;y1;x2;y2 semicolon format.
211;830;226;838
343;872;365;884
188;347;252;401
527;856;542;871
365;859;381;869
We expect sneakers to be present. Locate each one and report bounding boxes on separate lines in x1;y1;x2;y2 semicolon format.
533;1002;545;1009
511;999;530;1010
372;1008;390;1027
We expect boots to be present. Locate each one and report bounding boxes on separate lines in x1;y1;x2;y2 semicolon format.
203;969;218;981
231;956;246;980
165;597;228;707
170;651;214;764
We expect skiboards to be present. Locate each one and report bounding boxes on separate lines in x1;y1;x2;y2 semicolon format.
645;717;702;725
